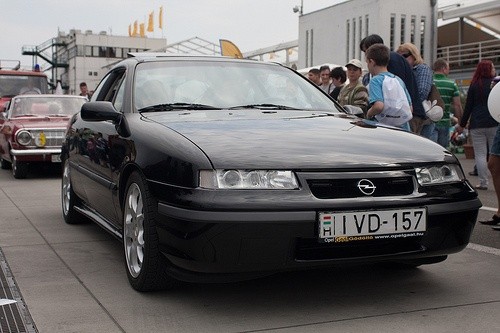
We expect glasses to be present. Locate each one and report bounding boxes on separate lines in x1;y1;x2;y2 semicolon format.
402;52;412;59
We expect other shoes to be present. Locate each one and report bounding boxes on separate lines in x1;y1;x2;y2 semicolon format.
478;214;500;231
475;185;488;191
469;171;478;176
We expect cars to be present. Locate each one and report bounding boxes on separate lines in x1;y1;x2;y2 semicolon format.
59;51;483;294
0;93;90;180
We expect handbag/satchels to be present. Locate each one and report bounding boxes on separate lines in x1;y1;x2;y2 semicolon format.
421;83;445;123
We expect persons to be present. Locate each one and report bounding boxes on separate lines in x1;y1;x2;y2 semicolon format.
33;64;95;102
305;33;500;231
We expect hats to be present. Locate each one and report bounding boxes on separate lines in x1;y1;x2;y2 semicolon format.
345;59;363;68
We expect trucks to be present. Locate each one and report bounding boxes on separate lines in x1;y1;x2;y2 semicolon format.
0;62;50;113
